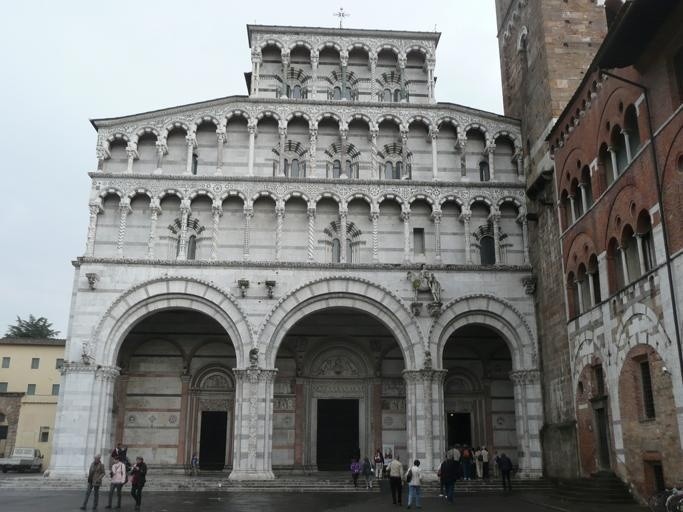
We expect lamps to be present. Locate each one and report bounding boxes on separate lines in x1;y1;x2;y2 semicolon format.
264;280;276;298
522;276;536;294
427;303;443;314
85;273;100;289
238;280;249;298
411;301;421;315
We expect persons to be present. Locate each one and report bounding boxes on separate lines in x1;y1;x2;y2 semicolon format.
112;443;132;471
106;457;126;510
190;454;197;475
129;456;147;510
78;454;105;511
351;444;515;510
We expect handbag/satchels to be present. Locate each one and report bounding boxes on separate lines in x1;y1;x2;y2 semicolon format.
407;470;412;482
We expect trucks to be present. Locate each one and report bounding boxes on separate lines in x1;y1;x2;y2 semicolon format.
0;447;44;473
